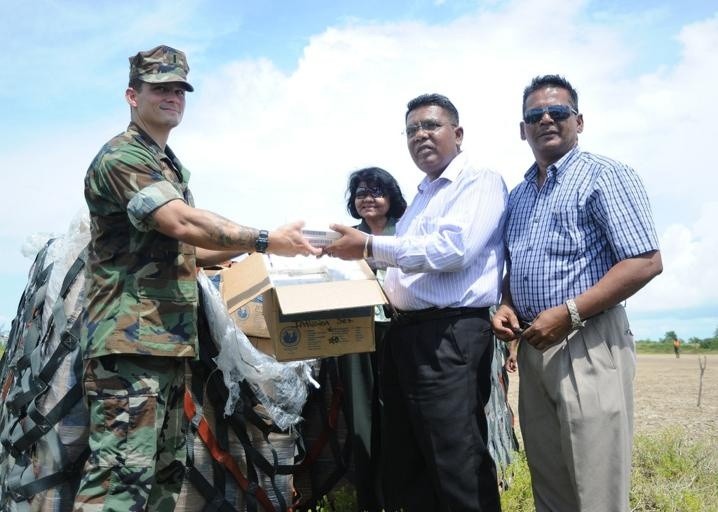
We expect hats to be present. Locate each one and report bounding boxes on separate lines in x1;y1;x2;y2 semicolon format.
129;45;195;93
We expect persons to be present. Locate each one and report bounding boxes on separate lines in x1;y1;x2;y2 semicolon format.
674;339;680;358
69;45;325;510
319;95;501;511
487;74;663;512
484;291;520;495
338;166;407;510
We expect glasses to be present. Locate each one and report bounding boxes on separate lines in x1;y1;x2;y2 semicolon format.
524;106;578;124
354;186;384;198
405;118;457;138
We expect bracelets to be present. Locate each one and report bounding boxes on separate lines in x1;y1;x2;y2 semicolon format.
363;232;373;263
568;298;585;330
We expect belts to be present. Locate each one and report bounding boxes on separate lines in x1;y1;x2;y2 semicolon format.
391;306;473;325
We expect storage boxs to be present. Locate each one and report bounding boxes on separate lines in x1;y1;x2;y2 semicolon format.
196;244;396;370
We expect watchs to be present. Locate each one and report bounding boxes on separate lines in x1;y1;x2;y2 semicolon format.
255;228;269;254
